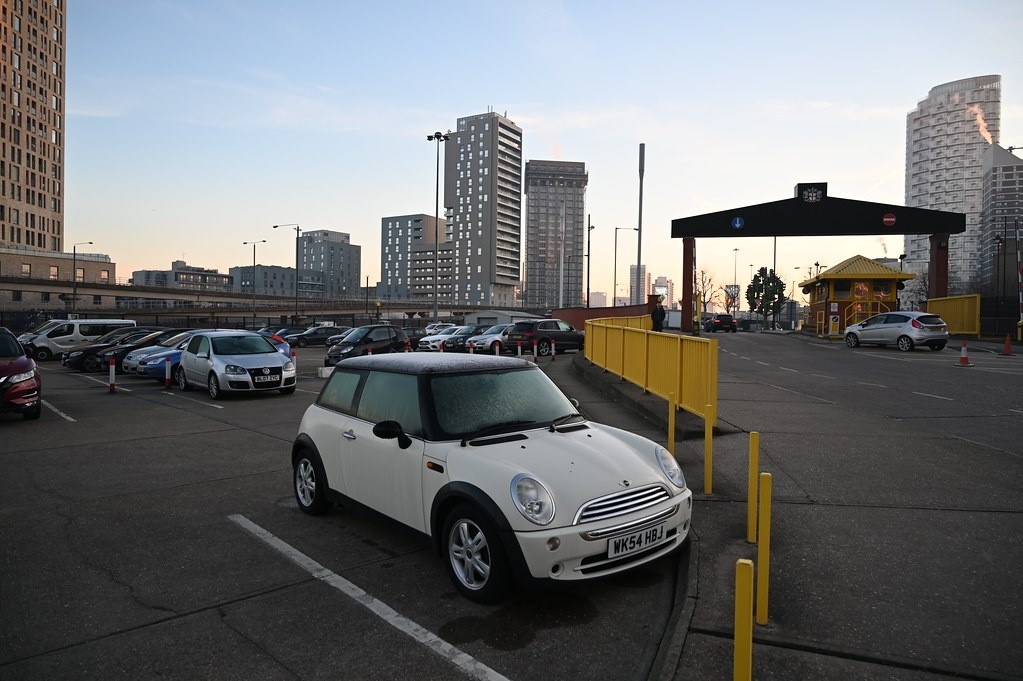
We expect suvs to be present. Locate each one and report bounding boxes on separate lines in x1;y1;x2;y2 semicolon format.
706;313;737;333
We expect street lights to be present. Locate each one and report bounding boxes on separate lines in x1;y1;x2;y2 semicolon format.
273;224;300;325
733;248;758;331
613;227;639;307
73;242;93;313
794;266;811;280
567;255;588;308
790;281;799;329
242;240;266;331
427;132;451;320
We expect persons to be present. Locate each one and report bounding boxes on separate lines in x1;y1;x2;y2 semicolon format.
650;301;665;332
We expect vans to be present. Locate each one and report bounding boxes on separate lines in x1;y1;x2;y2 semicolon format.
19;319;136;362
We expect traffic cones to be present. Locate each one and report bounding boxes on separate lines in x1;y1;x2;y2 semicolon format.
998;333;1017;356
952;341;976;367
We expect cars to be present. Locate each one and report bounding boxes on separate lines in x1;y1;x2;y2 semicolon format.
62;326;246;374
844;311;949;352
290;351;693;601
137;331;291;386
17;319;70;347
177;331;297;401
0;326;42;420
255;318;585;367
